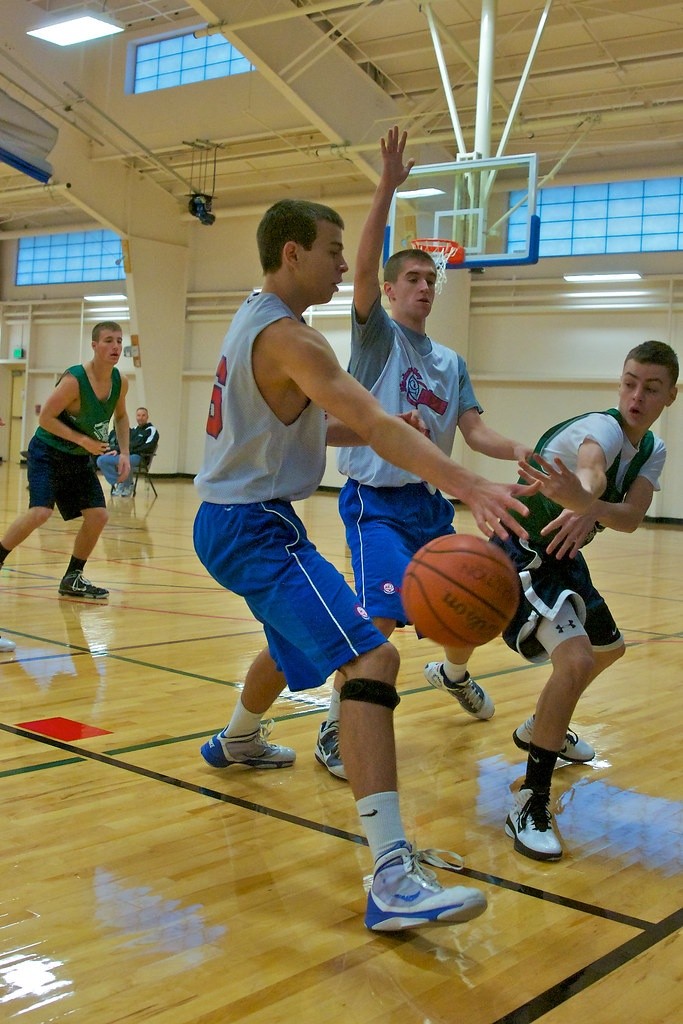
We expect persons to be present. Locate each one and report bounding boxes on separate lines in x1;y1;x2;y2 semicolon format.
97;406;159;497
1;321;127;599
315;125;535;782
490;340;679;863
193;197;531;932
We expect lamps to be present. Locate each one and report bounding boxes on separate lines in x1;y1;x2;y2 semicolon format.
182;139;226;224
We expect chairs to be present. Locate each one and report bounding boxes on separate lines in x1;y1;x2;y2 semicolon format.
110;445;157;497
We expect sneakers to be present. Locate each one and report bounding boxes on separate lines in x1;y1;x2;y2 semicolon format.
315;720;348;780
424;662;495;720
505;785;562;862
200;719;296;768
58;569;109;598
363;837;487;930
512;713;595;761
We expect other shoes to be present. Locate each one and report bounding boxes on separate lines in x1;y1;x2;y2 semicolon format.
112;482;123;495
0;635;16;651
120;481;134;496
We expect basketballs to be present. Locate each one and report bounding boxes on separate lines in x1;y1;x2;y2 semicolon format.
400;532;522;649
